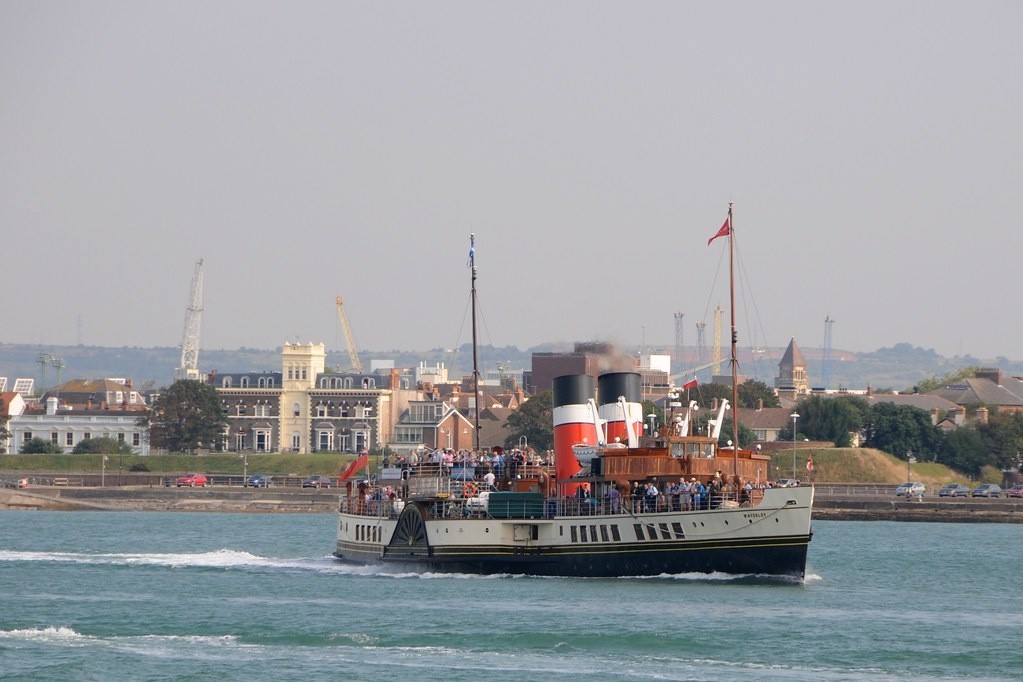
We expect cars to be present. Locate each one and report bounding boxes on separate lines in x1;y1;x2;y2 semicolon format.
775;478;797;488
895;481;927;497
972;483;1002;499
177;472;208;488
303;473;332;488
1005;485;1023;498
244;474;273;488
938;482;969;498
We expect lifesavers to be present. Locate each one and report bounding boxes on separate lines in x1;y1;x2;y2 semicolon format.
463;482;476;497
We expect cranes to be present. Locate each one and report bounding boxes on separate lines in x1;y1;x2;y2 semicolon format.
666;305;725;389
335;293;364;374
173;256;218;385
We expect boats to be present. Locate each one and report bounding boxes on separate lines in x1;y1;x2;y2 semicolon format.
332;233;816;585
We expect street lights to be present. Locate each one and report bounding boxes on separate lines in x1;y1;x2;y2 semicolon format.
101;454;110;486
789;411;801;480
239;453;249;486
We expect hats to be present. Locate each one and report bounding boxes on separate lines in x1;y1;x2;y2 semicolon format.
483;451;487;453
546;450;549;452
691;478;695;481
493;452;497;455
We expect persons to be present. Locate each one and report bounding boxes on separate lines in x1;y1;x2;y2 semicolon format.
484;469;495;491
382;447;555;468
573;472;781;513
363;486;402;515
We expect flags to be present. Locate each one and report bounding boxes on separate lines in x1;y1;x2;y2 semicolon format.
707;218;729;246
466;241;475;268
806;453;814;472
338;446;369;485
683;376;698;390
